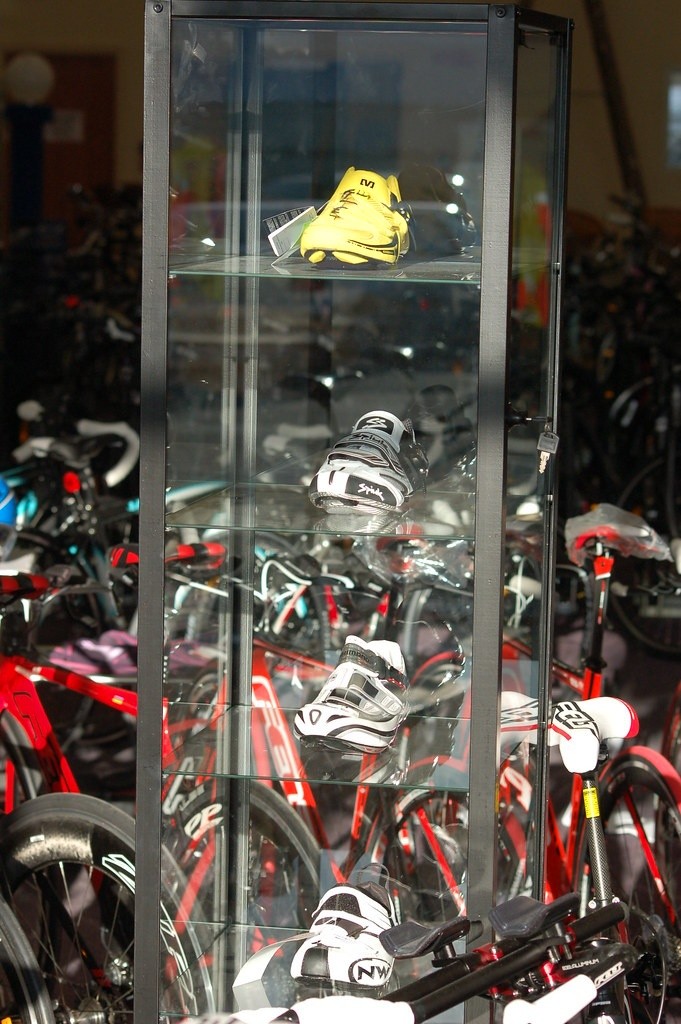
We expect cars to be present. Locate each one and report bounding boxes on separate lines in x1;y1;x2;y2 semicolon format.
169;159;479;259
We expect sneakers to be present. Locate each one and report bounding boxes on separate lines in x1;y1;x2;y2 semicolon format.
300;164;415;273
288;882;404;988
306;411;430;510
294;636;408;748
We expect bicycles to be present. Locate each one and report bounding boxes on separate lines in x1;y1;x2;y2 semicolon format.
3;346;678;1024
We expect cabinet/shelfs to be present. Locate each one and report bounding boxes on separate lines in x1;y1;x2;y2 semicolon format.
137;1;572;1023
